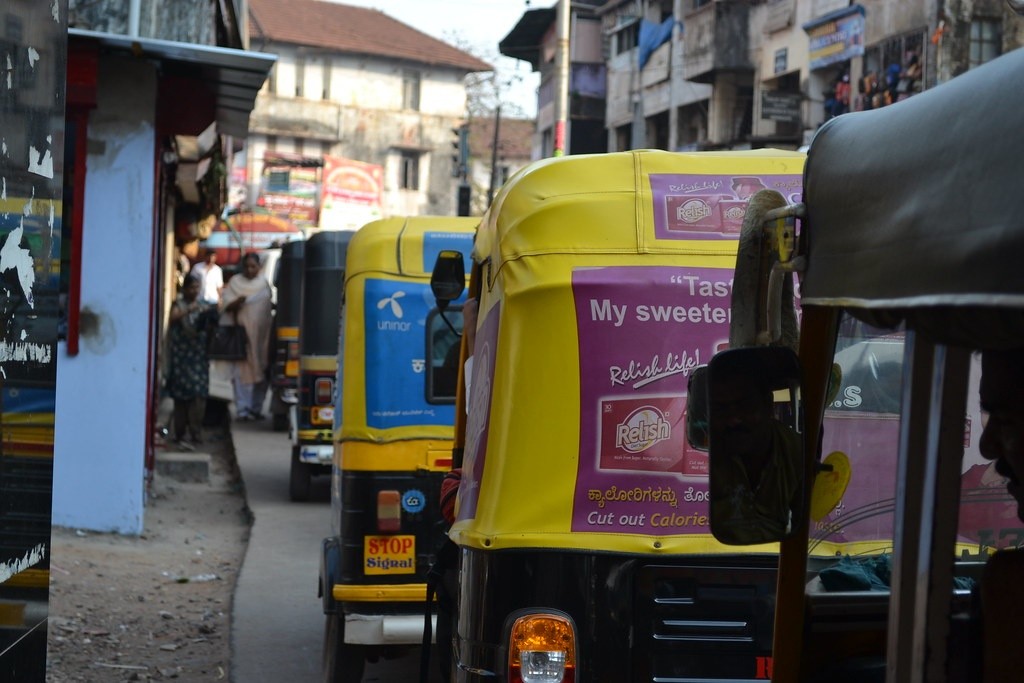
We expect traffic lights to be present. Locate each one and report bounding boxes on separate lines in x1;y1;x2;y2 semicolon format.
451;123;470;166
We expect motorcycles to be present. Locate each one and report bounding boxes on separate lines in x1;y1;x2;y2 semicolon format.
680;45;1024;683
292;231;356;499
322;217;485;681
420;142;1019;683
267;241;305;429
1;197;61;631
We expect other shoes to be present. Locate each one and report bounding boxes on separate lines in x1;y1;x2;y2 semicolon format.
176;434;186;445
192;436;203;445
250;411;265;419
238;416;248;422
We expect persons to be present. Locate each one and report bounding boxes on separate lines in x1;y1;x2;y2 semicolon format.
165;276;211;444
221;252;272;422
970;347;1024;523
190;249;224;306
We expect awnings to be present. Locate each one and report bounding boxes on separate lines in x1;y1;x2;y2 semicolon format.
67;26;278;140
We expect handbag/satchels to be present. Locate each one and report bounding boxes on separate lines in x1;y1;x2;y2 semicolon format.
206;310;248;362
